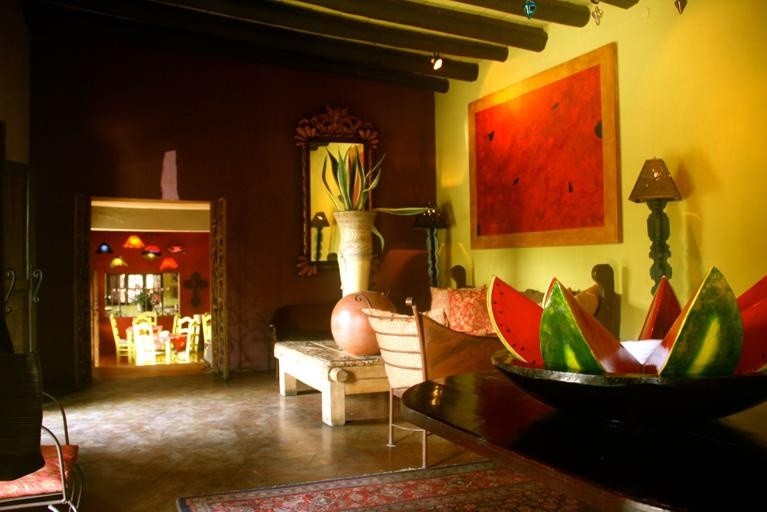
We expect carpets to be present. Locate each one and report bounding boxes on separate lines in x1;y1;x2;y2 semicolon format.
177;457;586;512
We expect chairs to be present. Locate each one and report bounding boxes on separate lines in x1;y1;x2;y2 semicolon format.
398;265;621;342
353;293;511;469
0;389;85;512
107;301;214;369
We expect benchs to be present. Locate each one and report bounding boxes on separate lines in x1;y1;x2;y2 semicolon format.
268;301;336;386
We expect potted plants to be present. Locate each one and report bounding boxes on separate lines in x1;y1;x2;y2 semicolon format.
129;290;156;311
318;141;431;297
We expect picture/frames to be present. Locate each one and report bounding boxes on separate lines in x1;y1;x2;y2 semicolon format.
465;42;625;249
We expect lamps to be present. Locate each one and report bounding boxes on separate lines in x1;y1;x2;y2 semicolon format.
310;211;331;260
96;233;184;272
411;207;451;287
629;157;681;295
427;49;444;69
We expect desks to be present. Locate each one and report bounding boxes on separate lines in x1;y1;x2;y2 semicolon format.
272;339;390;429
396;362;767;512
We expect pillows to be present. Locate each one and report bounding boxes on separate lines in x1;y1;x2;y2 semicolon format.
444;286;494;336
422;286;448;312
538;285;599;320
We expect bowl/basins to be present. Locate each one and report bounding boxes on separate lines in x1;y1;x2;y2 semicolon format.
490;340;767;436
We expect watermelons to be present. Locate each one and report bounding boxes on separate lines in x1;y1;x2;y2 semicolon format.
486;265;767;378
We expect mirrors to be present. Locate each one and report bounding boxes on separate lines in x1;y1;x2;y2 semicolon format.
287;101;382;278
103;271;182;319
